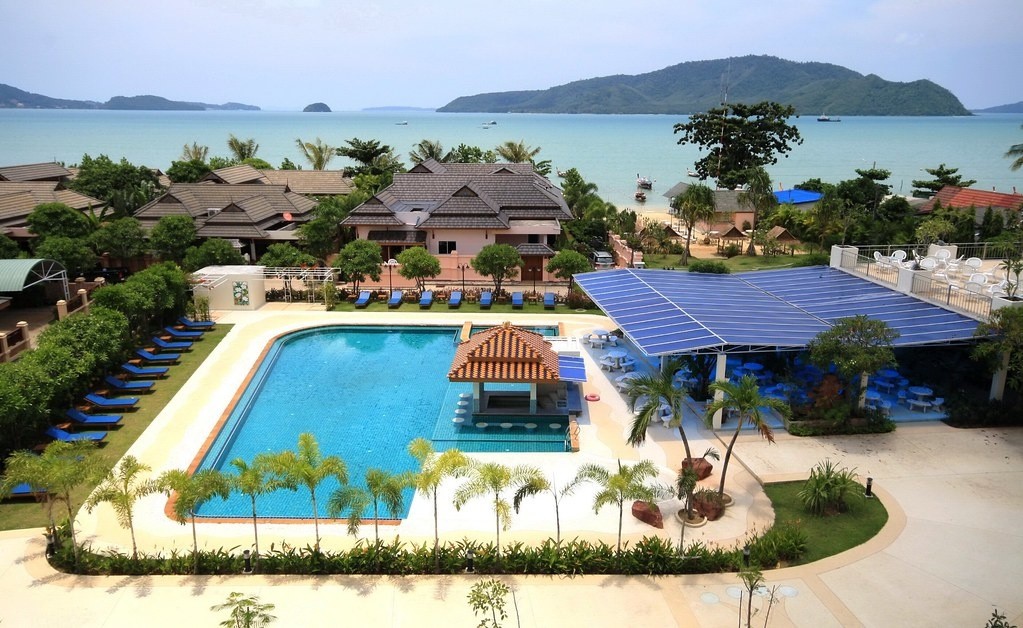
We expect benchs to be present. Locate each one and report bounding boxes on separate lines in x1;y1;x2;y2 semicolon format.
1;316;216;503
345;290;560;309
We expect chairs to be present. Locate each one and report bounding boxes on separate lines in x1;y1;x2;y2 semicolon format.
584;208;1023;430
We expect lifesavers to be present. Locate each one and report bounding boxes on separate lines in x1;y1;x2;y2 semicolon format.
585;393;600;401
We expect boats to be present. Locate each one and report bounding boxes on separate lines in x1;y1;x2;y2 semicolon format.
555;166;568;178
635;190;647;202
635;172;652;190
817;113;841;122
686;168;701;178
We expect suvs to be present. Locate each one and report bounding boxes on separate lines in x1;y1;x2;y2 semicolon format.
69;265;133;286
587;250;616;271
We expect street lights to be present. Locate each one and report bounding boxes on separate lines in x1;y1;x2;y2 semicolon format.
384;263;396;300
456;263;471;292
528;263;542;296
